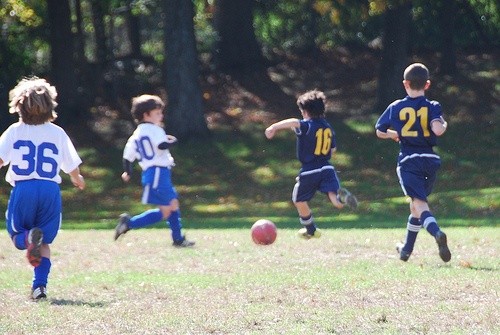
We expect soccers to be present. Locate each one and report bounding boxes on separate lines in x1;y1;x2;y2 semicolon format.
250;219;277;245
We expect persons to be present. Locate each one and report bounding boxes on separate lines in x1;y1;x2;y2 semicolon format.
114;94;196;247
265;88;358;238
375;63;451;262
0;75;85;302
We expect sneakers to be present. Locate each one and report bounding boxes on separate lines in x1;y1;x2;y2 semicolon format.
29;228;44;268
114;213;131;241
435;232;452;263
397;241;411;262
32;287;47;299
173;240;196;247
299;227;320;239
337;187;358;207
